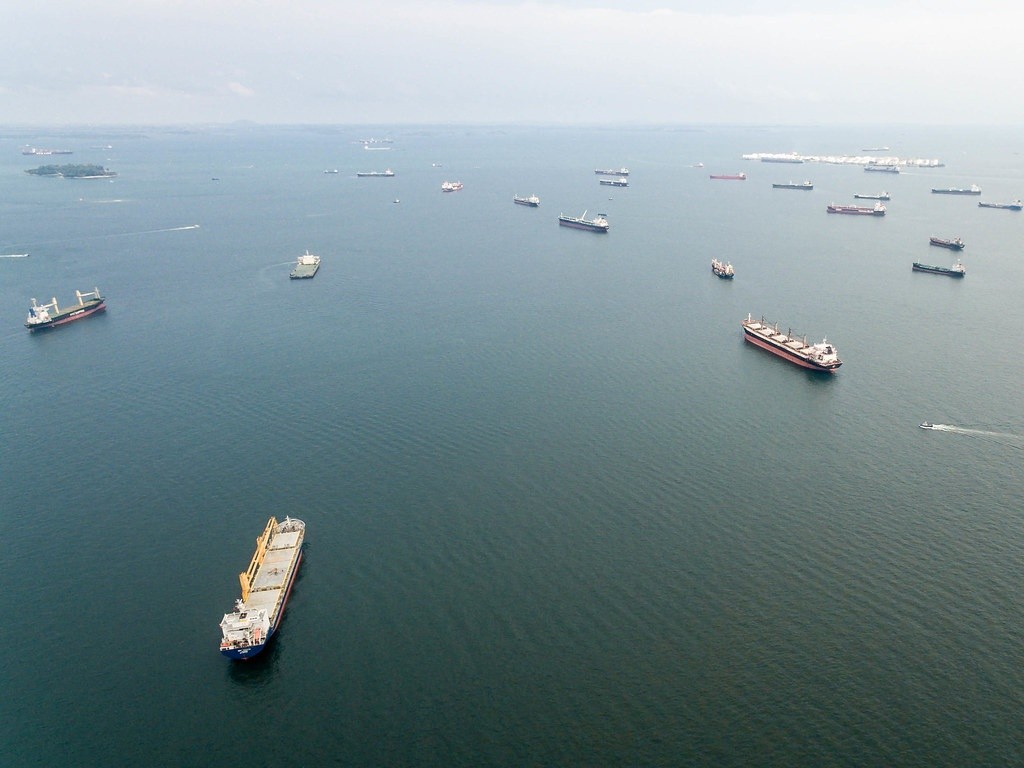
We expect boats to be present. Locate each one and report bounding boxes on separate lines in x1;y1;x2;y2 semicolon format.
22;145;73;155
931;184;981;195
219;516;306;660
325;169;395;176
289;249;320;279
979;200;1024;210
513;193;541;207
772;178;814;190
855;192;890;200
595;168;629;187
741;313;843;371
23;286;107;333
919;421;934;429
930;234;965;249
762;147;901;172
442;180;464;191
912;258;966;275
827;200;888;217
558;210;610;232
712;259;735;279
